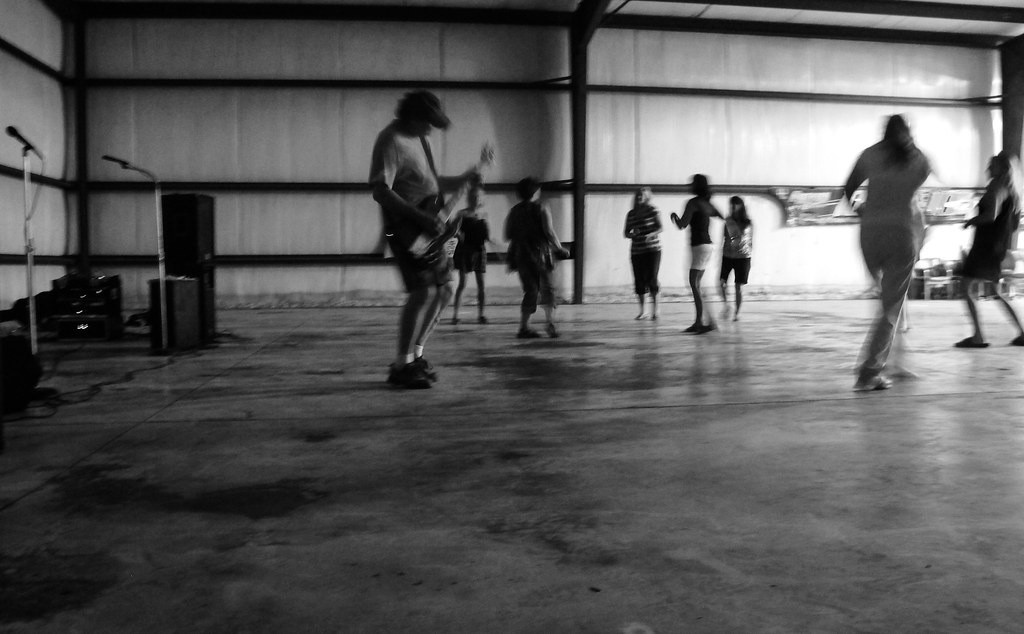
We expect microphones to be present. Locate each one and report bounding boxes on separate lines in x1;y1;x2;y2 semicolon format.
6;126;41;159
102;155;129;165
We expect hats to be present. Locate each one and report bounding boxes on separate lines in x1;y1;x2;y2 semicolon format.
517;178;538;199
399;92;451;131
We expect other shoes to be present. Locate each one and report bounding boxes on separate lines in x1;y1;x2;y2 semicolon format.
732;315;739;321
853;376;892;392
545;323;559;337
684;324;716;334
651;314;660;320
477;315;488;324
635;313;649;320
451;319;458;325
517;327;541;339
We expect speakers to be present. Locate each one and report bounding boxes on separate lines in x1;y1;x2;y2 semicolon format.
160;192;218;345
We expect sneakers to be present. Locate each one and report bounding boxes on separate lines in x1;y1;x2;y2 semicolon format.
386;357;437;390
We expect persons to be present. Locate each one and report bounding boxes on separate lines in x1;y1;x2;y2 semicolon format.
370;88;495;389
953;156;1024;347
625;187;662;321
502;175;570;341
846;117;934;394
670;175;724;337
715;196;753;322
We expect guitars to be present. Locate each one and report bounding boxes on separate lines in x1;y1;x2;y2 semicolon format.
407;143;495;267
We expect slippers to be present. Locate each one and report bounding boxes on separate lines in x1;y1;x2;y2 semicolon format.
1010;335;1024;346
956;338;989;348
